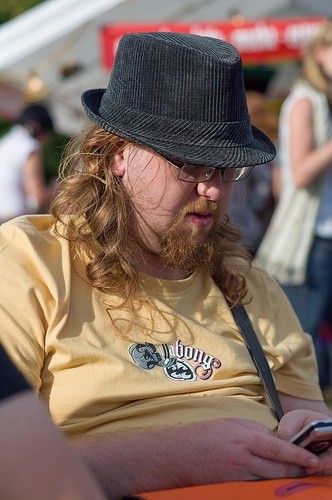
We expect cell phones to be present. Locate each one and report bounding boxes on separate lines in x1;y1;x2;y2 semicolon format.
288;418;332;455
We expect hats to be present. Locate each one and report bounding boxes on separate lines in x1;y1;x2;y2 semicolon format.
79;31;277;168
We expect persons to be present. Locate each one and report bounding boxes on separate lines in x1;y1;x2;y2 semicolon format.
0;104;50;222
277;19;332;389
222;91;277;260
0;345;109;500
0;31;332;497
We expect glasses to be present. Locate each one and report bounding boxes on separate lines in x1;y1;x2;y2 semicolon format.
157;154;254;183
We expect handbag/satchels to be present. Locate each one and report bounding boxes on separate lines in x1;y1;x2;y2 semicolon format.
250;185;320;283
